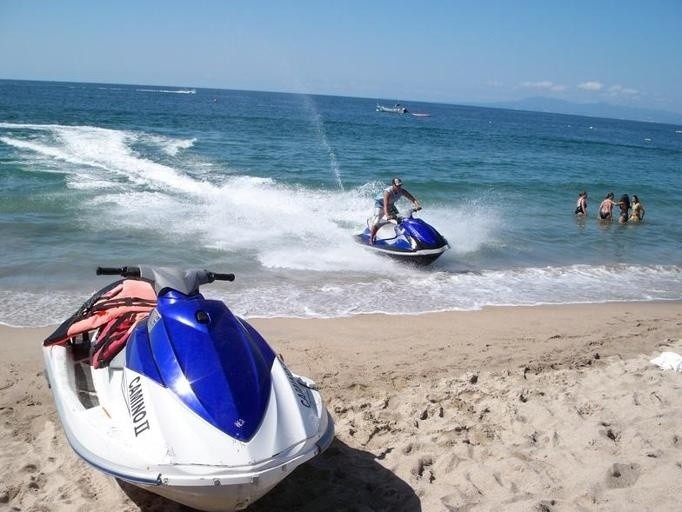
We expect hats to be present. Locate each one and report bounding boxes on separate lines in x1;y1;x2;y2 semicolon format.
392;178;403;187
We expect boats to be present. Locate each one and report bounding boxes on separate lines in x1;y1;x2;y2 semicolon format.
41;263;335;510
375;104;407;114
412;112;432;117
351;207;451;265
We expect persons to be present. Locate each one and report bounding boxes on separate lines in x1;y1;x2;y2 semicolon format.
597;191;614;221
607;194;631;224
573;190;589;216
367;177;422;246
575;215;638;233
626;195;645;223
